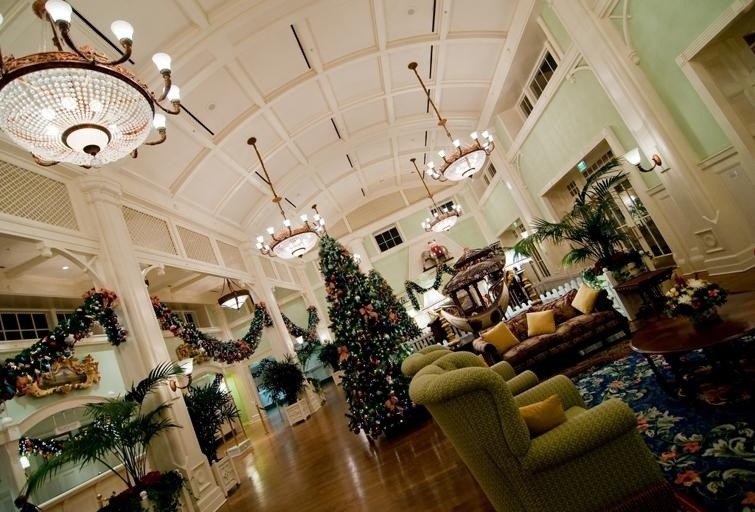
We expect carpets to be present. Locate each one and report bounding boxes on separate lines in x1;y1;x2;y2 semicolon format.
569;339;755;510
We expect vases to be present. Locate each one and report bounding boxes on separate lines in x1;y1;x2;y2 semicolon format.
579;255;659;321
686;304;721;329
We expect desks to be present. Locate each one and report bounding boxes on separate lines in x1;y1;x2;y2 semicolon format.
629;316;754;401
612;269;675;321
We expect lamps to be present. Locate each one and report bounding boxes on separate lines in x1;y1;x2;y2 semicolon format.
169;357;194;391
218;277;252;312
245;134;327;262
408;156;463;237
408;59;498;183
623;144;663;176
0;0;181;170
19;454;35;479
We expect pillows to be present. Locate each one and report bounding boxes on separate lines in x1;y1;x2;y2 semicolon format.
511;389;569;427
480;320;522;353
571;282;600;316
524;309;558;337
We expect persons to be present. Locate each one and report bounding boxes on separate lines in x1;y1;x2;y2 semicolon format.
14;496;41;512
505;271;516;281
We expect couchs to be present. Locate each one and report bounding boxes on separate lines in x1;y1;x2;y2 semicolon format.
399;339;538;397
469;285;631;373
407;351;677;511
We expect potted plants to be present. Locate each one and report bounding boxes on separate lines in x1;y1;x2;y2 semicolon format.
256;358;311;428
177;383;245;499
293;347;328;406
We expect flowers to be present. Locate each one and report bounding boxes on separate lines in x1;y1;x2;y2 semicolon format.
18;360;190;512
512;154;647;288
662;271;729;320
